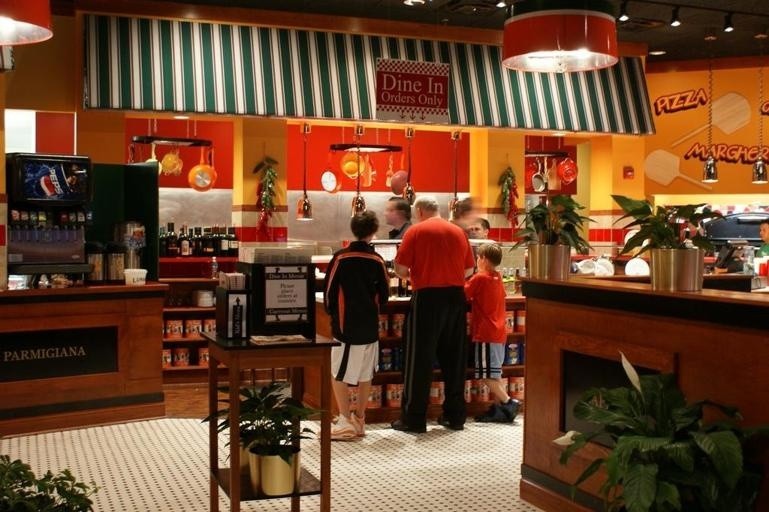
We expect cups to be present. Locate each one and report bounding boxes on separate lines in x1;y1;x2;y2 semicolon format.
87;221;144;279
759;259;769;277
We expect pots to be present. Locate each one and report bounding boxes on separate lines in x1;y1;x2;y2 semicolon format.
145;145;163;179
531;155;545;193
340;150;373;189
186;145;218;193
390;150;409;195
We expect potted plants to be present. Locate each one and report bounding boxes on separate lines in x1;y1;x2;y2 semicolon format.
509;194;599;281
200;380;330;496
611;194;727;292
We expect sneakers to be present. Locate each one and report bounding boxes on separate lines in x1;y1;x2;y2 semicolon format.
474;398;521;423
319;413;365;440
437;413;464;430
391;419;427;432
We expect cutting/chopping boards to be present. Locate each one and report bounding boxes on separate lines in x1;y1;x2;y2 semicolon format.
547;158;562;192
644;148;713;192
670;91;751;149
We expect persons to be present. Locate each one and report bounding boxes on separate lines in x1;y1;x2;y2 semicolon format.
463;243;522;423
448;197;475;240
754;219;769;257
393;195;476;434
467;215;491;240
319;210;391;443
384;196;413;240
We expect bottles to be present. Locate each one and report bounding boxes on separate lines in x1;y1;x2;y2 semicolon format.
209;256;218;280
385;261;411;298
158;222;240;258
499;266;527;297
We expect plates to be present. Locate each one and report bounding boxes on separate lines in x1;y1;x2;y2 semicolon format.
623;229;650;248
193;289;215;307
595;258;615;277
625;257;650;276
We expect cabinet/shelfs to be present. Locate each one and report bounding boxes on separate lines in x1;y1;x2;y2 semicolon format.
159;257;241;383
302;294;527;423
199;330;341;512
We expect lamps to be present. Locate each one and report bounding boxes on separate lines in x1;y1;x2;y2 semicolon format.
449;130;460;213
502;0;620;73
350;127;366;218
702;33;720;182
619;2;769;32
296;124;313;220
752;35;769;184
0;0;54;46
403;127;416;206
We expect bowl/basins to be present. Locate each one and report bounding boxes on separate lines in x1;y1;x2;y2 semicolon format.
123;268;147;285
